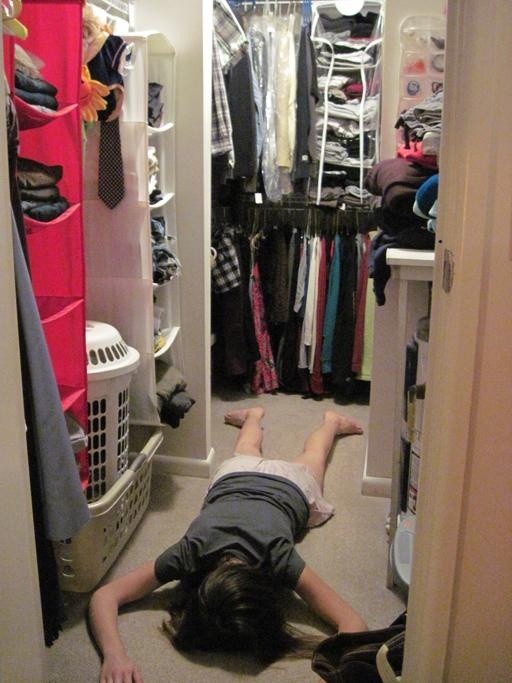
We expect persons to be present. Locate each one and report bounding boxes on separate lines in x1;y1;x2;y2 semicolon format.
90;407;369;683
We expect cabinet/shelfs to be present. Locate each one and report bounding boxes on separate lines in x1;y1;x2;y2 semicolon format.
0;0;94;503
87;29;181;429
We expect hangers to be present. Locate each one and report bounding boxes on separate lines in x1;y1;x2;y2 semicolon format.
238;3;312;28
211;197;378;238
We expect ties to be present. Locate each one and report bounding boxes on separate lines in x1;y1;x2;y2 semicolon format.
98;117;124;210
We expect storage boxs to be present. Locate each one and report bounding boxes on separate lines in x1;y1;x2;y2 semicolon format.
55;422;168;596
